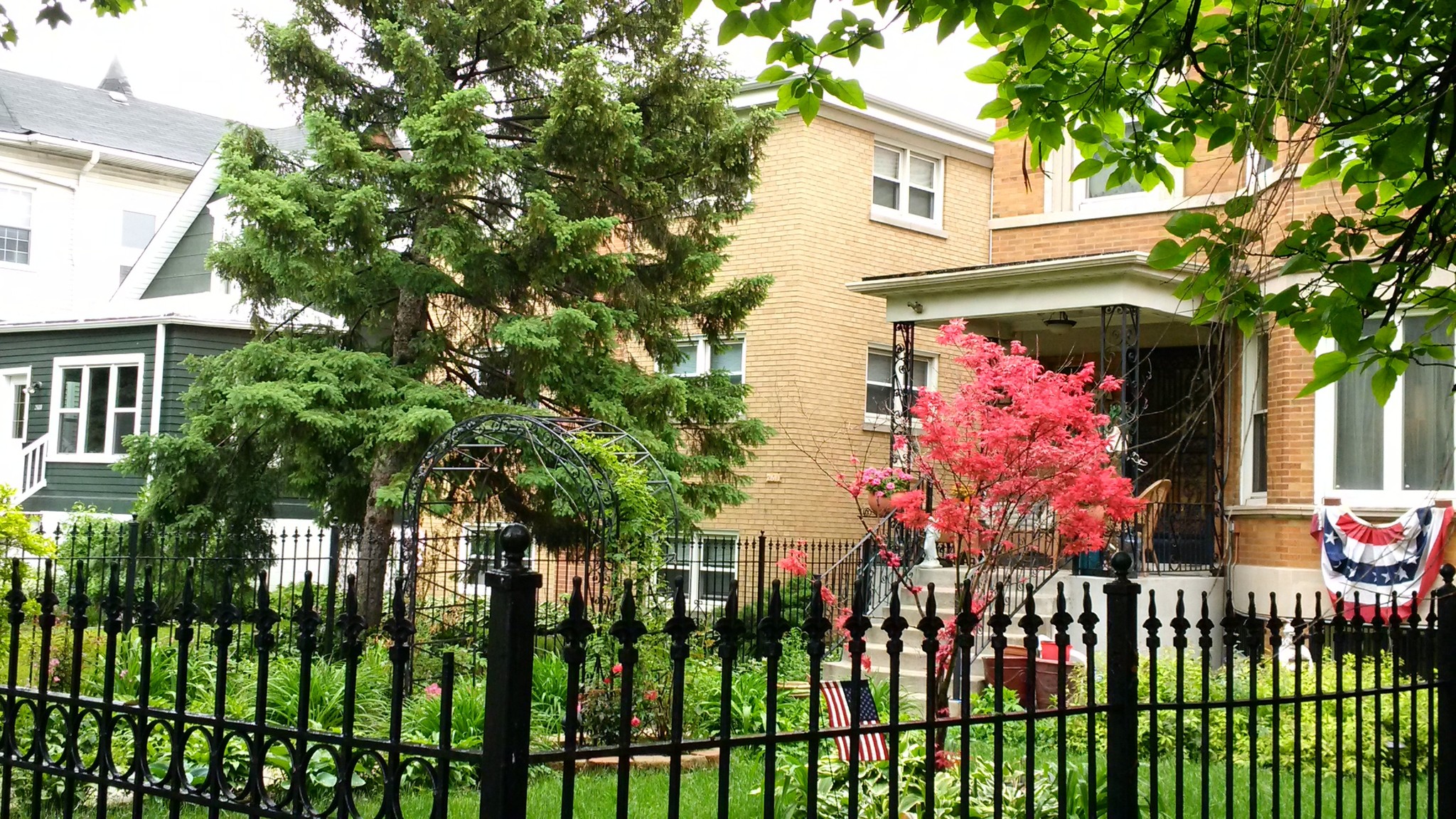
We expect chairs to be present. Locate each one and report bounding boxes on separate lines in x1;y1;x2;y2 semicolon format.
1032;478;1172;577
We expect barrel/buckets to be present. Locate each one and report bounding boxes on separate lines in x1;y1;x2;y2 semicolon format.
1041;641;1073;661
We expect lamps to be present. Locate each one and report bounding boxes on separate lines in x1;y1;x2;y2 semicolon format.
1043;311;1078;337
22;381;43;393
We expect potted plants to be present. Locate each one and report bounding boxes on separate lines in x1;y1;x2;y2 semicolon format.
776;626;812;699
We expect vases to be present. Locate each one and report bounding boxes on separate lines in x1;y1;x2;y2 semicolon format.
981;655;1083;708
868;491;909;514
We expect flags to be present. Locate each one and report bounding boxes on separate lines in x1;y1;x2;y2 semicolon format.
820;679;889;762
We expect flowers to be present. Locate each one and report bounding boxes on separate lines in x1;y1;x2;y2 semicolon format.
862;467;915;498
